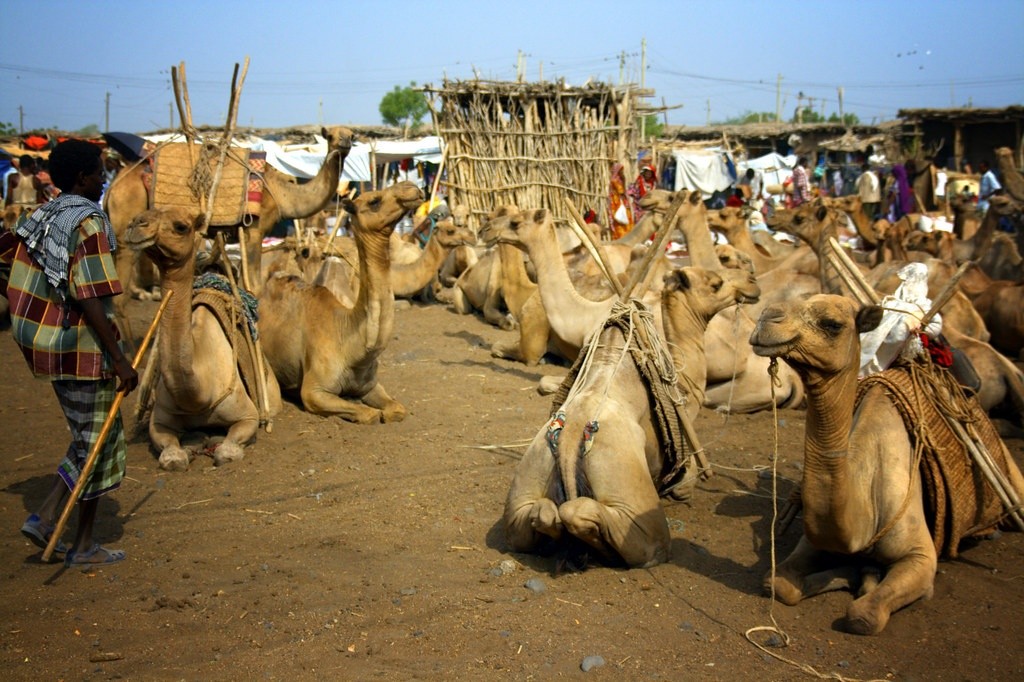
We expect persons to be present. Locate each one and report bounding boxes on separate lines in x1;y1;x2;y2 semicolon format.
584;209;609;241
0;138;138;567
660;148;1001;225
630;164;660;227
605;162;633;242
411;204;452;305
0;150;126;215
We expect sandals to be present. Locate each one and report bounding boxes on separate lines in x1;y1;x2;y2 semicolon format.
61;544;127;568
22;514;68;553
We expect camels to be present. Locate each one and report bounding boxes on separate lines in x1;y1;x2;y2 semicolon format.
745;294;1024;635
506;263;762;568
105;126;1008;473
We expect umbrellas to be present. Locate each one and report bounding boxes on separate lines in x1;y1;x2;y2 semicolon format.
100;132;151;164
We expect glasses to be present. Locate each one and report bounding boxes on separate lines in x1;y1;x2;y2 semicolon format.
96;169;106;179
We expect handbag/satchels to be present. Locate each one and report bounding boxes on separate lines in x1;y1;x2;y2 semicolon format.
614;197;629;225
